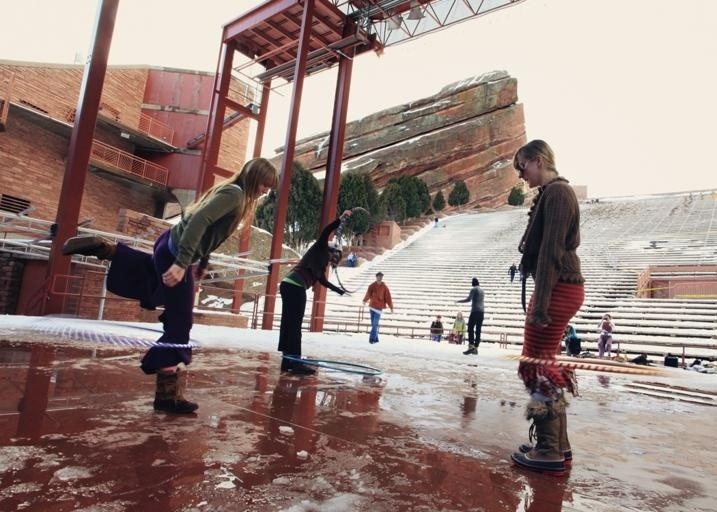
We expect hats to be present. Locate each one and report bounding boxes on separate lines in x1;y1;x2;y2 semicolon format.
328;243;343;268
472;278;479;286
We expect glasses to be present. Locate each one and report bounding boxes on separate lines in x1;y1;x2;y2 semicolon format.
516;157;537;174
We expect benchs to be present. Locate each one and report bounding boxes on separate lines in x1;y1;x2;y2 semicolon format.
239;194;717;365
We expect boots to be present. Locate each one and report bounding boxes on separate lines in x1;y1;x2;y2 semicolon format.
62;236;116;261
153;367;199;413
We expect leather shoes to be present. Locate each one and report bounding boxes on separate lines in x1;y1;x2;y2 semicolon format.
510;393;572;476
281;358;315;373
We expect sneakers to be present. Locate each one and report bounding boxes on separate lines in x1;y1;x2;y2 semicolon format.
463;345;478;355
369;338;378;344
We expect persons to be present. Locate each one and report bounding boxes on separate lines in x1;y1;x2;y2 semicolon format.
429;315;444;342
347;252;353;266
690;359;702;367
508;263;517;282
560;323;579;357
454;276;485;355
510;140;586;472
62;157;279;416
351;251;357;266
453;312;464;345
361;272;393;343
596;313;614;357
629;351;656;367
444;327;460;345
276;210;352;376
662;353;678;367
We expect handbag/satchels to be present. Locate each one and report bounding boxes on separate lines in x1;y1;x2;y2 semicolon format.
568;338;580;354
